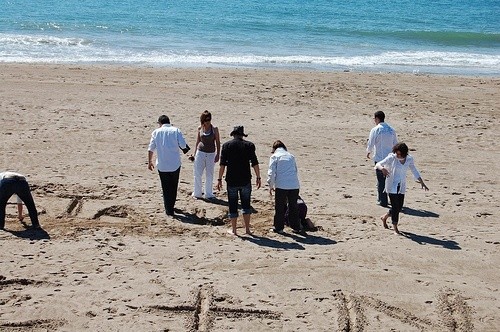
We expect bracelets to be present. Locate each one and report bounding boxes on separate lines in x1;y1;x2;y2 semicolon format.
257;177;261;180
189;154;193;157
218;179;221;181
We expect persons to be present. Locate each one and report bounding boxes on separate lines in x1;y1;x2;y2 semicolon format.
191;110;220;201
0;172;41;230
285;191;313;230
366;111;398;206
147;115;195;217
375;142;429;234
216;125;261;235
267;139;301;234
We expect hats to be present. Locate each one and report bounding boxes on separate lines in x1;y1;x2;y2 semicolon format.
230;126;248;138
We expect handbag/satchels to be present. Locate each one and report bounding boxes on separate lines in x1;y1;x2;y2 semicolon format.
285;198;307;227
